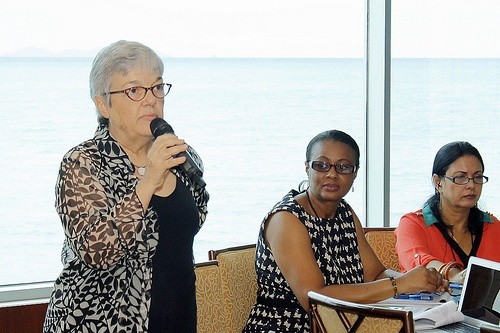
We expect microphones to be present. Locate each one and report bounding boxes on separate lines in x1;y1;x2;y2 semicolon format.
150;117;203;181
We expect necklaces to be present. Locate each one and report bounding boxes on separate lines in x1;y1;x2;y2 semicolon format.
305;190;319;218
450;228;474;250
134;163;146;176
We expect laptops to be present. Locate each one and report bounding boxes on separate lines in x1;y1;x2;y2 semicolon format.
414;256;500;333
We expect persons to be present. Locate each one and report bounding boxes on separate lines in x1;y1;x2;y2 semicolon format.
394;141;500;284
242;130;452;333
43;40;209;333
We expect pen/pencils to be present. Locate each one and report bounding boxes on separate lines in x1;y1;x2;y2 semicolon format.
450;283;462;289
398;294;433;300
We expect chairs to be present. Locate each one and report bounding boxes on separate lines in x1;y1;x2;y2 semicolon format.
193;228;414;333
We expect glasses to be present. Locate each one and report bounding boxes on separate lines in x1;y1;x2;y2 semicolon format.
308;160;358;174
101;83;173;102
444;175;489;185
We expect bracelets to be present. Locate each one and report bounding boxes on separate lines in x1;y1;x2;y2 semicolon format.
442;260;464;280
437;262;446;274
389;276;398;299
